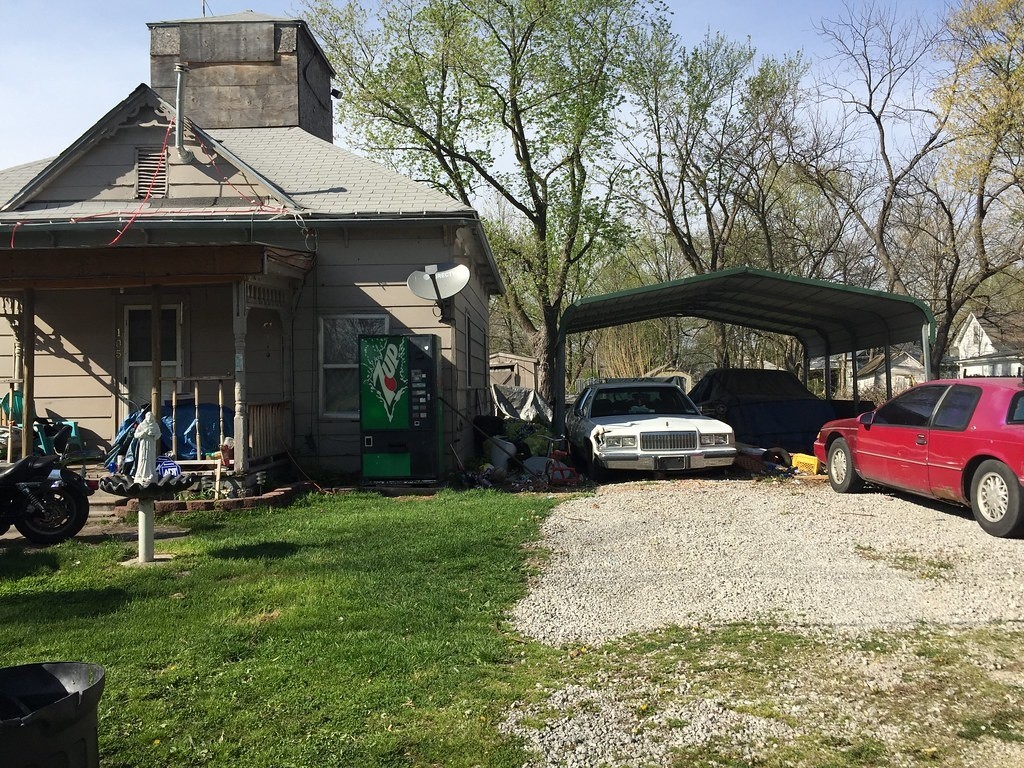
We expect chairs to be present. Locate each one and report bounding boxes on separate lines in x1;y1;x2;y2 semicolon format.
0;389;80;455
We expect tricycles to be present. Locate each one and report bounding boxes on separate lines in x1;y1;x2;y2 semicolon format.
536;433;586;486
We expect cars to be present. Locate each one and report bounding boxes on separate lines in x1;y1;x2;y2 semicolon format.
812;376;1024;541
565;378;737;481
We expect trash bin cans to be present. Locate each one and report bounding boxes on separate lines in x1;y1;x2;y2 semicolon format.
0;661;107;768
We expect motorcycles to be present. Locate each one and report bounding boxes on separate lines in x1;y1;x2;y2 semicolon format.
0;453;100;548
